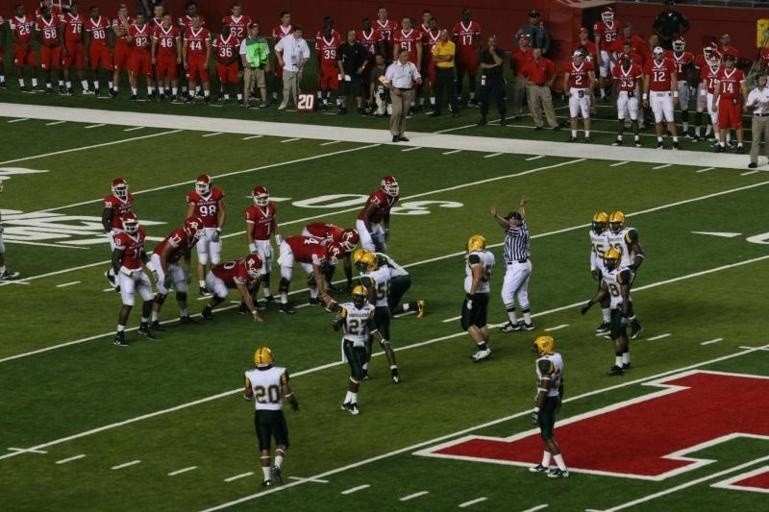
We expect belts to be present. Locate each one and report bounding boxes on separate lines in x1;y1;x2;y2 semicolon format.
507;260;527;265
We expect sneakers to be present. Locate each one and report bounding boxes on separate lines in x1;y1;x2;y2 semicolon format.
237;294;323;316
529;463;550;474
260;480;274;491
113;330;131;347
476;117;489;126
501;119;508;126
197;287;214;298
340;405;362;417
501;322;523;335
748;162;758;168
104;272;116;290
629;325;642;341
137;322;167;341
179;314;198;326
521;323;537;332
201;309;217;320
271;464;285;485
606;363;634;376
547;469;570;480
595;321;616;341
1;270;21;281
416;298;427;320
470;345;493;362
570;132;744;152
392;135;410;142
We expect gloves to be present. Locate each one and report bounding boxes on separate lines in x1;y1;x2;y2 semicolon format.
531;407;540;426
466;300;473;310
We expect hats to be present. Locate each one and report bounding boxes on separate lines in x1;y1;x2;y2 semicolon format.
572;50;584;59
653;47;666;56
505;212;522;221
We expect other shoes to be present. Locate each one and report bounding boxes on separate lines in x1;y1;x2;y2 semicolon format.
0;86;120;101
133;94;268;111
278;94;479;119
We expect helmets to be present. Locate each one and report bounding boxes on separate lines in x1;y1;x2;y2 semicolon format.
341;228;360;253
381;175;399;198
195;174;212;194
111;178;129;197
122;212;141;233
604;247;623;268
465;233;487;254
361;251;378;271
591;211;608;232
328;242;344;262
251;186;270;207
354;248;367;265
601;6;615;22
185;217;204;240
352;285;368;301
608;211;626;234
246;253;263;278
254;348;275;368
533;335;555;356
671;36;721;68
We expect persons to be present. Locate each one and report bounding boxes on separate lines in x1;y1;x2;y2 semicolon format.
563;2;769;169
314;7;560;142
459;235;496;363
243;348;301;487
332;285;392;416
582;210;645;377
529;335;570;479
490;194;536;332
1;2;311;113
103;173;423;347
